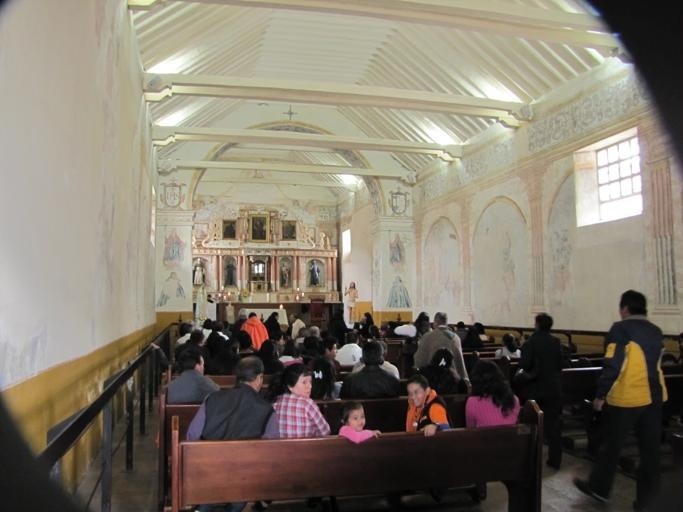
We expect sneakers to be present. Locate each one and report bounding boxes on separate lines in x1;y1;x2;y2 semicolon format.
573;477;609;503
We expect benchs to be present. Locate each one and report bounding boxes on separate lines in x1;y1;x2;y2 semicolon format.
158;337;683;512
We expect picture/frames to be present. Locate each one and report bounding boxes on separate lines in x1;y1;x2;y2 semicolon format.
247;214;270;243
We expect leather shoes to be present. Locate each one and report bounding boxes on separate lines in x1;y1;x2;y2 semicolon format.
546;458;560;471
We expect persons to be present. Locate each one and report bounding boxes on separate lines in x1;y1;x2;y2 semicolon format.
159;311;572;512
344;281;359;325
661;350;679;376
193;220;322;285
570;289;668;510
